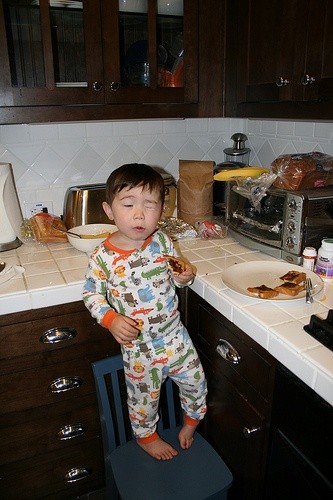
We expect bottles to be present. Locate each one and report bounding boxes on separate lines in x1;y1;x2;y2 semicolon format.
160;173;177;218
315;238;333;279
303;247;318;271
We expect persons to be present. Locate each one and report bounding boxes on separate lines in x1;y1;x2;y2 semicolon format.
81;164;207;460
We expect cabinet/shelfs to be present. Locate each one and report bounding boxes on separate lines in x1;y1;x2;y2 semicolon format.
0;0;225;126
0;298;180;500
223;0;333;121
177;286;333;500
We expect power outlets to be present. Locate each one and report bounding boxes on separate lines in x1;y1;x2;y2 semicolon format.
24;201;53;219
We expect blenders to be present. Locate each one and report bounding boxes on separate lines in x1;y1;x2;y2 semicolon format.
213;133;251;214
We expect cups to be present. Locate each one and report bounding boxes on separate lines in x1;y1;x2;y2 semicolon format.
140;62;150;87
159;70;171;87
156;63;164;86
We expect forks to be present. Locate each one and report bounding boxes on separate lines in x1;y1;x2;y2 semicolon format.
304;278;313;304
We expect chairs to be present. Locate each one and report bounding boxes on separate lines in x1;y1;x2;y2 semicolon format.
90;354;234;500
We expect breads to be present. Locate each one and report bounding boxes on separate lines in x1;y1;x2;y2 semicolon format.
274;282;305;296
163;255;186;276
30;213;68;244
272;152;333;190
247;285;279;299
279;271;306;284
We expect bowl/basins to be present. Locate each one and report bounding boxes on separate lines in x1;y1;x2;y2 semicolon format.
65;223;118;253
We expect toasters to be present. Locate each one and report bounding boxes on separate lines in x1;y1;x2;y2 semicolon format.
62;183;116;229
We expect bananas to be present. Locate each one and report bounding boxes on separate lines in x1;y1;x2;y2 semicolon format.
213;168;269;181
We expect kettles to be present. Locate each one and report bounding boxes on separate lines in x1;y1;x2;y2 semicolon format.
0;162;24;251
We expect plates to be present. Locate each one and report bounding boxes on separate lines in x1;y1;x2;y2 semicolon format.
221;261;323;301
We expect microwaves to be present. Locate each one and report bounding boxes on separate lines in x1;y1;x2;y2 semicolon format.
224;181;333;264
